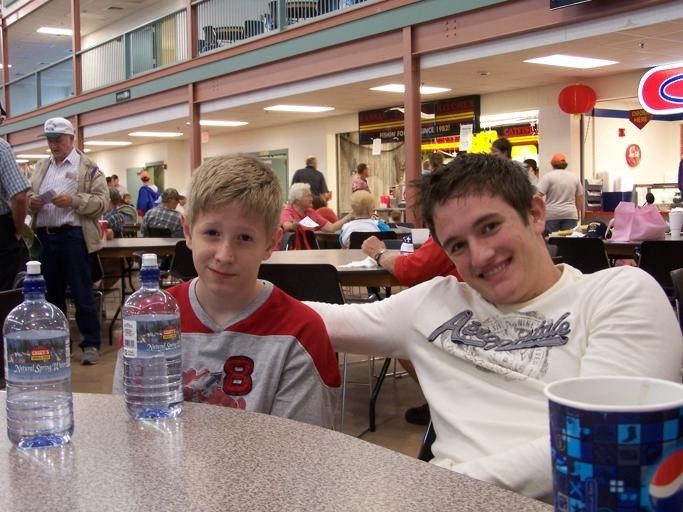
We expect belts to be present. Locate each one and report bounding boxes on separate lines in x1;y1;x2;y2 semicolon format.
37;227;67;236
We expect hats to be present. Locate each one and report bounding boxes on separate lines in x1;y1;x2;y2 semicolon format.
36;117;77;139
551;154;567;167
159;188;185;201
138;171;149;179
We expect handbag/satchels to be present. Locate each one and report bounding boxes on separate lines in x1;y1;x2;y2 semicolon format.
601;202;669;243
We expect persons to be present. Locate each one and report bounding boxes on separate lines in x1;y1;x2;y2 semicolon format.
111;174;129;198
300;153;683;504
339;188;391;249
537;153;585;233
136;170;162;218
429;153;444;170
140;187;185;238
351;162;371;192
28;117;110;366
0;103;32;291
123;193;134;207
98;187;125;239
274;182;354;251
112;153;342;429
312;197;338;224
491;138;512;160
360;235;465;288
291;157;332;201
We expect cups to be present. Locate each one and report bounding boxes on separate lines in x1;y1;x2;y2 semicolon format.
669;213;683;238
544;375;683;512
411;228;430;249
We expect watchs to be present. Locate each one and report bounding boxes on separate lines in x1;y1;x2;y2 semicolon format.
374;250;385;265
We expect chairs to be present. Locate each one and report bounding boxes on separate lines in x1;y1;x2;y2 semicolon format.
146;225;171;238
632;240;682;326
202;25;220;50
245;20;265;39
556;237;611;274
350;231;397;250
258;263;347;305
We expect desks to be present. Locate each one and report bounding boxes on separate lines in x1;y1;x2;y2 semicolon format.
313;225;412;249
102;237;185;346
262;249;406;432
0;390;555;511
546;226;683;294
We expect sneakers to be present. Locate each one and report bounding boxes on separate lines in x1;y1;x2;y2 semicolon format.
80;347;101;366
404;400;431;427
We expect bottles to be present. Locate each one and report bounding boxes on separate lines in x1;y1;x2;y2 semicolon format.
2;260;74;452
121;253;185;422
